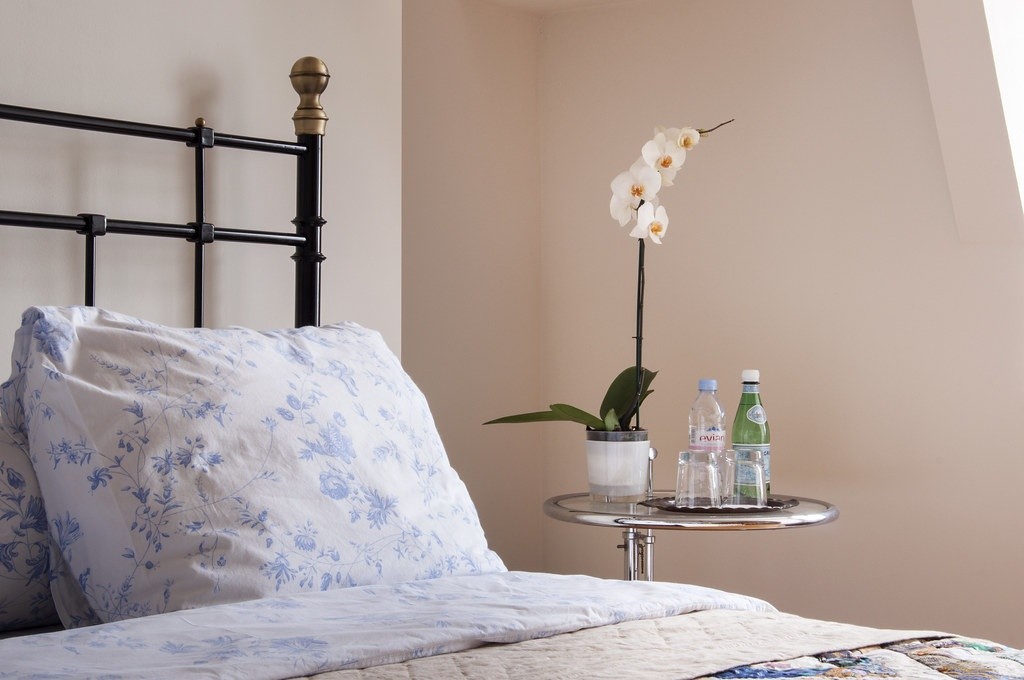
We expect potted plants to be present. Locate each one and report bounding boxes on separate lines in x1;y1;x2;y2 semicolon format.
482;365;660;505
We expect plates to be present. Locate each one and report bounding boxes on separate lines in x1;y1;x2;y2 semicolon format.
636;497;800;511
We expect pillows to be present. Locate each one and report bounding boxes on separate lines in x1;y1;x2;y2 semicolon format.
0;303;507;632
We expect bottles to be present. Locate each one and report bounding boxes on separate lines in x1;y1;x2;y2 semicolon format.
731;369;771;496
688;379;727;462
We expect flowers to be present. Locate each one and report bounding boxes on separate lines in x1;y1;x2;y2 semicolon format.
486;117;735;430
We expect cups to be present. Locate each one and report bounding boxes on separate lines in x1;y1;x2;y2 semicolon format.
722;448;767;506
676;449;721;506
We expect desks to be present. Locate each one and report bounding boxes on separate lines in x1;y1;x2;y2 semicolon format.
545;495;840;580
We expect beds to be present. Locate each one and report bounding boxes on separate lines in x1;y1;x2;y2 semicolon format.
0;52;1024;680
